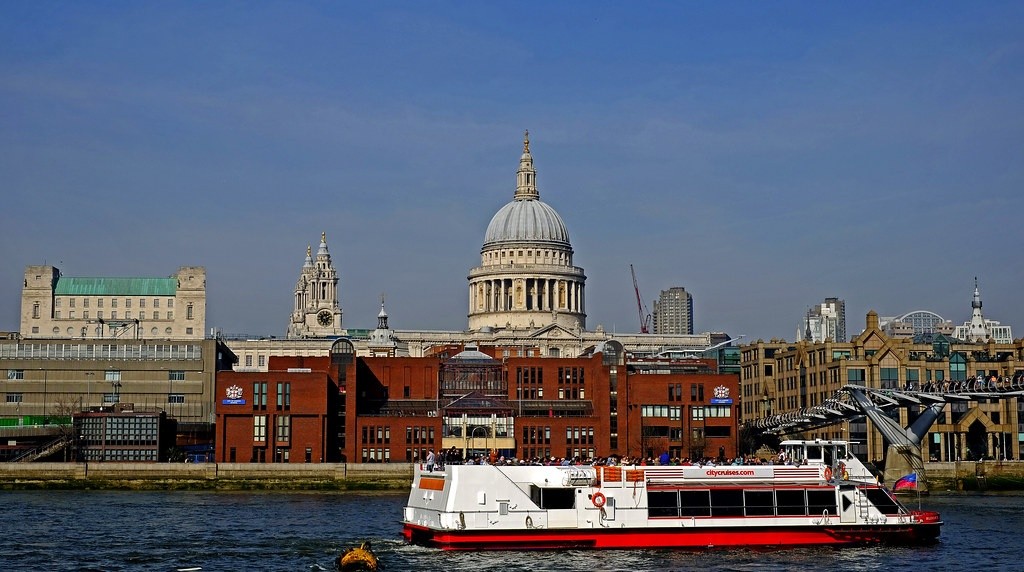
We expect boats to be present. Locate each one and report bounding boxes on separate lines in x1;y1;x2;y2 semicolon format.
398;438;944;553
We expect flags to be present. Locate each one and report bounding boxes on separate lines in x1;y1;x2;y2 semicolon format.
893;474;917;491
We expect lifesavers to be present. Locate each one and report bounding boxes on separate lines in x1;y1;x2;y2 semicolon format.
592;492;606;507
835;463;845;480
824;467;832;481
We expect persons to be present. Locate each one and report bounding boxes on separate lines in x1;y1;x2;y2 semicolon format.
471;453;618;466
446;446;462;465
902;371;1024;393
426;450;435;472
620;449;808;466
436;449;445;469
719;446;725;459
